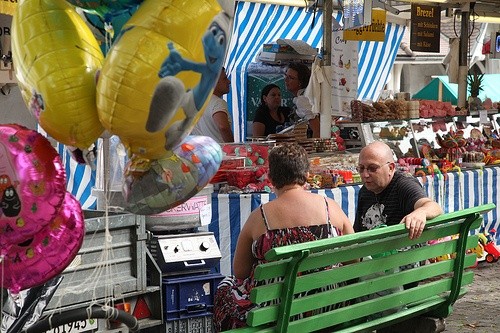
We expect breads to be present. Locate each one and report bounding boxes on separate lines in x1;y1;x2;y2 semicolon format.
349;99;419;120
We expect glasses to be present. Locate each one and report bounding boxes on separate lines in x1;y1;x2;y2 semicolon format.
356;162;390;173
284;74;299;80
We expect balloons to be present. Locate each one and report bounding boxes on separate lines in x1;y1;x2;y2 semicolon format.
0;124;67;256
11;0;235;214
0;192;84;293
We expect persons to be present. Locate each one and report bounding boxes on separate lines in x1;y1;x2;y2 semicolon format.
266;63;320;139
212;142;360;333
191;67;234;142
353;142;443;290
254;84;292;139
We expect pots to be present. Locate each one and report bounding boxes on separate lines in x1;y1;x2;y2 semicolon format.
144;184;214;236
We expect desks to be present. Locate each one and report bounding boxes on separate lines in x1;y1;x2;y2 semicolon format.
209;163;500;287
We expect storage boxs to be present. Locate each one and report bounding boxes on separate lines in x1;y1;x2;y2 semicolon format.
412;77;458;104
42;209;147;311
162;273;226;333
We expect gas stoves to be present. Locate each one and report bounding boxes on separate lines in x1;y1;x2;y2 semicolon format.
146;230;225;275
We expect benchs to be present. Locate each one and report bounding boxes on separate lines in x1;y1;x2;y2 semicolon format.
222;203;495;333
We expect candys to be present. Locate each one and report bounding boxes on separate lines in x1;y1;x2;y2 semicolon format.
422;126;493;163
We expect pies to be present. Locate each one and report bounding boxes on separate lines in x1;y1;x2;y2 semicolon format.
266;133;314;154
